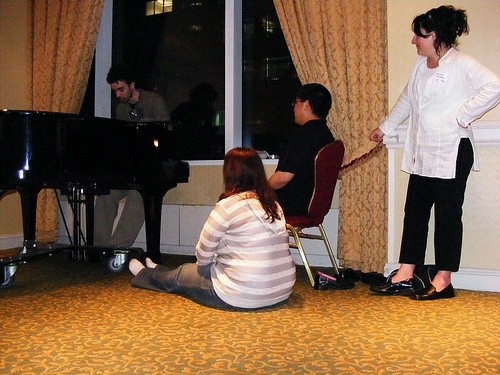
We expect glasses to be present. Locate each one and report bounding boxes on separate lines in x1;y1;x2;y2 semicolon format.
291;100;305;106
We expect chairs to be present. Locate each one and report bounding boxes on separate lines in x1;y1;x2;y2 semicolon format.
282;139;346;289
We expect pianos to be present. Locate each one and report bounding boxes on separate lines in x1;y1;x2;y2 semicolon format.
1;108;191;272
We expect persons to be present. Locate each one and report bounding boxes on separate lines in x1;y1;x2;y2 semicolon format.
369;6;500;300
123;147;295;310
92;62;170;249
216;83;334;218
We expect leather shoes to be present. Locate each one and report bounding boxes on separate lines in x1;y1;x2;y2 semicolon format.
369;278;415;294
411;283;455;300
313;270;355;290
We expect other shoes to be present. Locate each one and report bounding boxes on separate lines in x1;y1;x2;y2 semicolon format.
359;271;386;285
